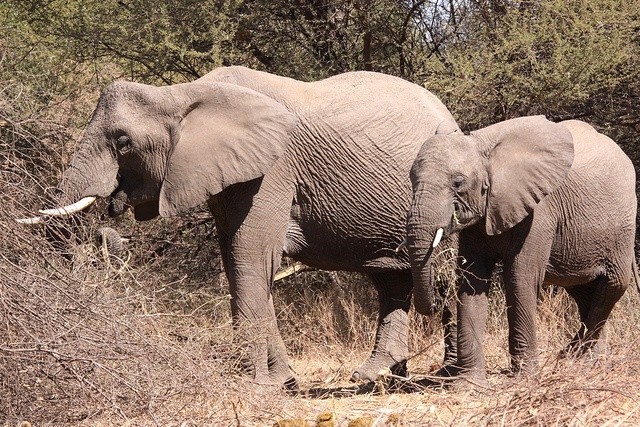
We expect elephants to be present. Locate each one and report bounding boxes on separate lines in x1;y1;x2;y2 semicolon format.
13;65;466;392
406;114;640;393
